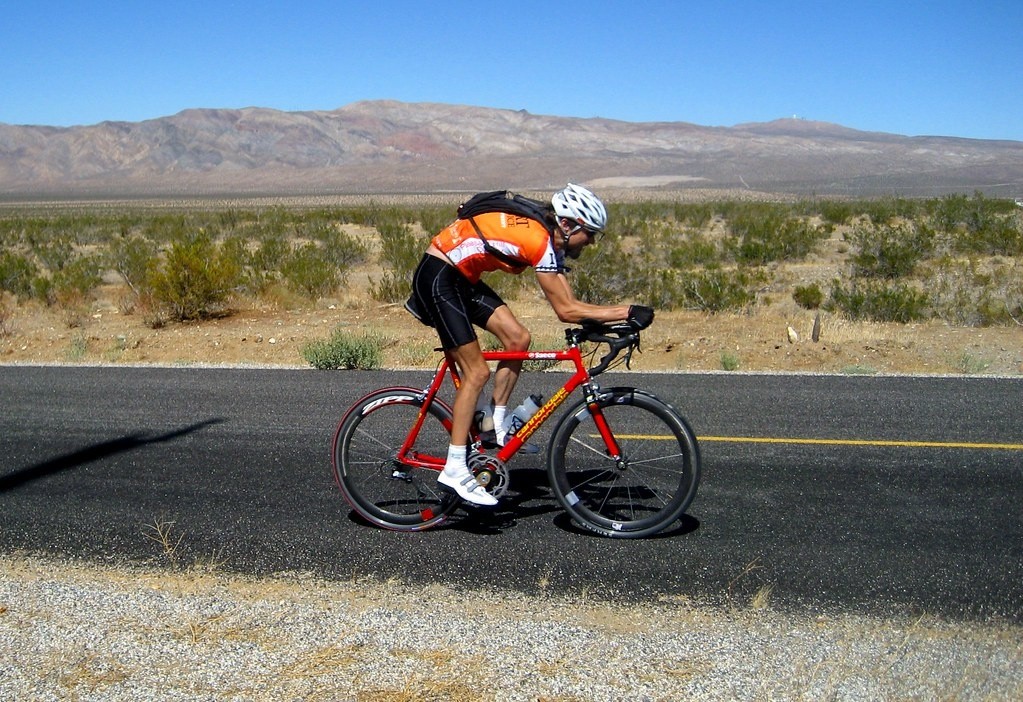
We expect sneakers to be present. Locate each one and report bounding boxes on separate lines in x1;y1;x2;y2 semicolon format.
436;470;498;507
480;423;539;453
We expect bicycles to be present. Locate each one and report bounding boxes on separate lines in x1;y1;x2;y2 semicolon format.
330;304;701;540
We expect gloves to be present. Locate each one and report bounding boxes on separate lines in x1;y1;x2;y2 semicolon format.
627;307;654;332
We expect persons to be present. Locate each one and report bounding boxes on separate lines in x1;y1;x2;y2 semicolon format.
413;183;655;506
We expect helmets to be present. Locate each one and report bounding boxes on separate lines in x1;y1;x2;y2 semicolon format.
551;182;607;228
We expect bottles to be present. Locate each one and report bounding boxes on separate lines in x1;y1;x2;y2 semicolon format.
476;388;497;449
500;393;544;434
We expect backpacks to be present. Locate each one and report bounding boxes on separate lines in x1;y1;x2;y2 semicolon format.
456;190;555;267
